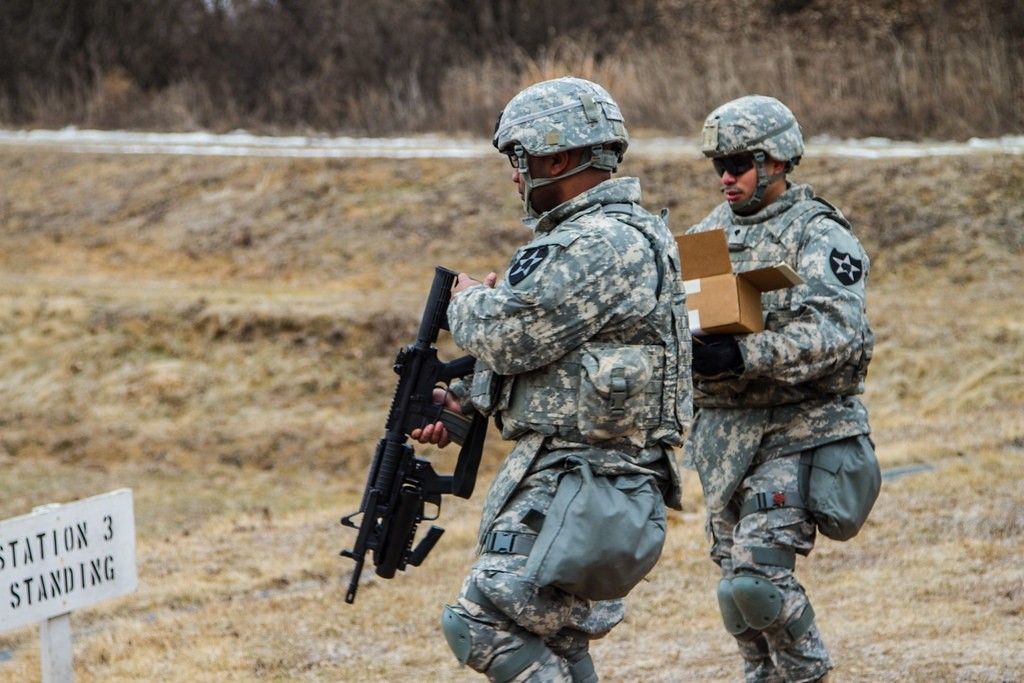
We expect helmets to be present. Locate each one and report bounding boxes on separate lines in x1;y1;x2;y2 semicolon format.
702;94;804;161
493;77;629;163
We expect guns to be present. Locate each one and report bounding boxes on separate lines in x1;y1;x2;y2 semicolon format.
341;267;500;605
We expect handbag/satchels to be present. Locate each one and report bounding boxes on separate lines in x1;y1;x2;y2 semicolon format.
809;431;882;542
523;459;668;599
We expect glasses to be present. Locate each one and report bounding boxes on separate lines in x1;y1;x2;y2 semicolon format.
710;152;755;177
506;147;521;168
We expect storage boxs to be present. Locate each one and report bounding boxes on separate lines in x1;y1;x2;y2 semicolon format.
673;228;803;338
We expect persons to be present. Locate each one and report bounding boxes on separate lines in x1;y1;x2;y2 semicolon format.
679;95;881;683
409;78;693;683
